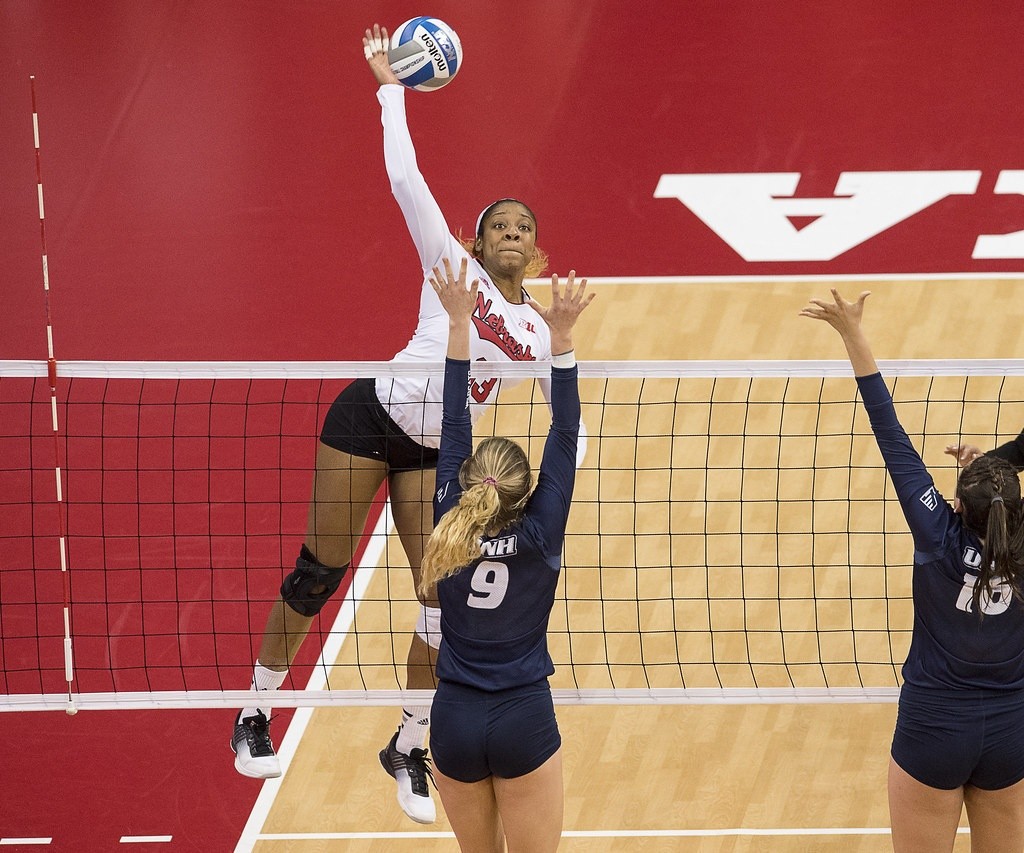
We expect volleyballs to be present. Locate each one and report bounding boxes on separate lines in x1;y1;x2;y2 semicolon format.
388;16;463;91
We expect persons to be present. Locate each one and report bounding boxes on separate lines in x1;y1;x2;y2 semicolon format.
230;25;589;825
417;256;596;853
798;288;1023;853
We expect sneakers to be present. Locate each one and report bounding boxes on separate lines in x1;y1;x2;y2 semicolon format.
230;709;282;778
379;725;438;825
